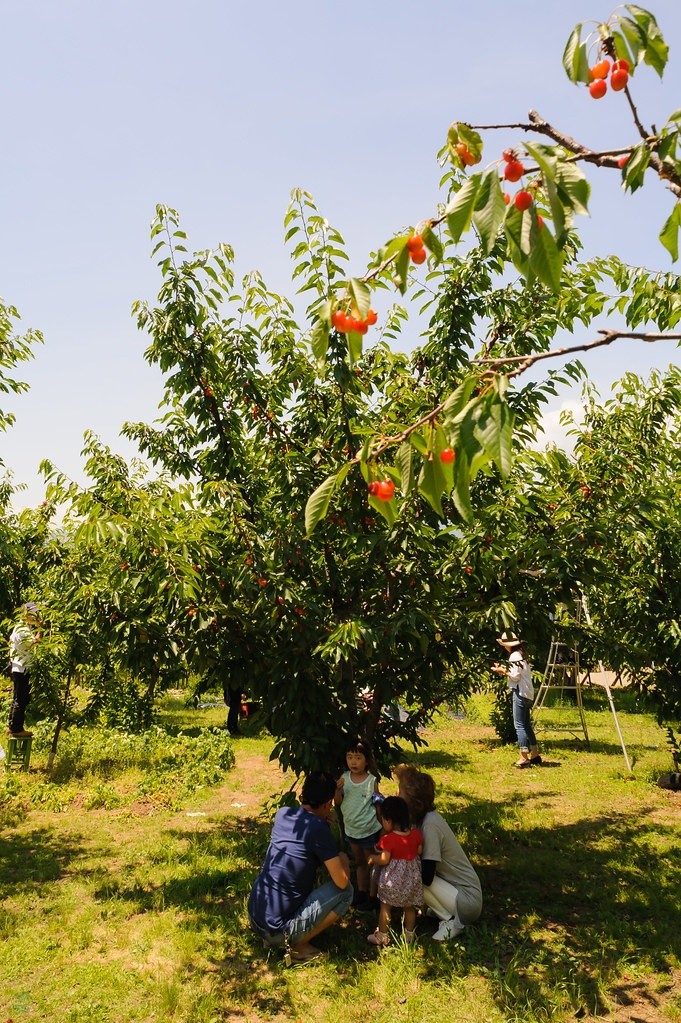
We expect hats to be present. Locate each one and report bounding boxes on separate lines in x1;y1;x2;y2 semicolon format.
496;631;522;646
24;603;42;612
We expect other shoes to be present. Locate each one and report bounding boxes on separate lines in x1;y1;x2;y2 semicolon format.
367;928;389;945
286;945;330;964
405;928;414;942
350;891;379;911
7;727;34;738
529;756;541;764
512;760;531;768
263;943;280;955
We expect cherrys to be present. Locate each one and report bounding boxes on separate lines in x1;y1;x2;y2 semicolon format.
188;32;631;643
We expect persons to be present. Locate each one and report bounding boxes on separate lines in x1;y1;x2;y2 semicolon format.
367;796;423;944
248;769;354;964
397;767;482;940
492;631;542;769
334;739;381;914
8;600;41;736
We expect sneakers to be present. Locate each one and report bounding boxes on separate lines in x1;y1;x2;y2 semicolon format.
432;918;463;941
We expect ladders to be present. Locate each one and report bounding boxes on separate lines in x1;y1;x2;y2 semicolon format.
522;596;592;751
